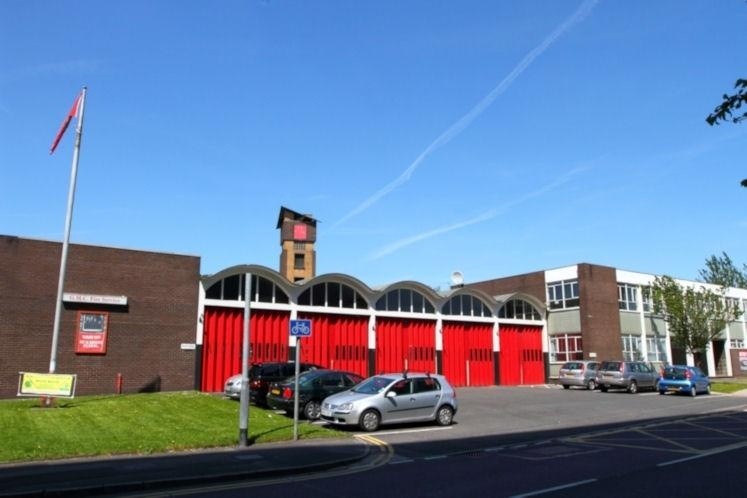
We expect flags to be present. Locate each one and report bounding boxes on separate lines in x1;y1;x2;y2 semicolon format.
49;90;82;155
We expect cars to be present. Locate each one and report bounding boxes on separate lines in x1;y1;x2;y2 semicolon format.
319;372;458;432
657;364;712;397
222;358;370;421
557;359;663;394
